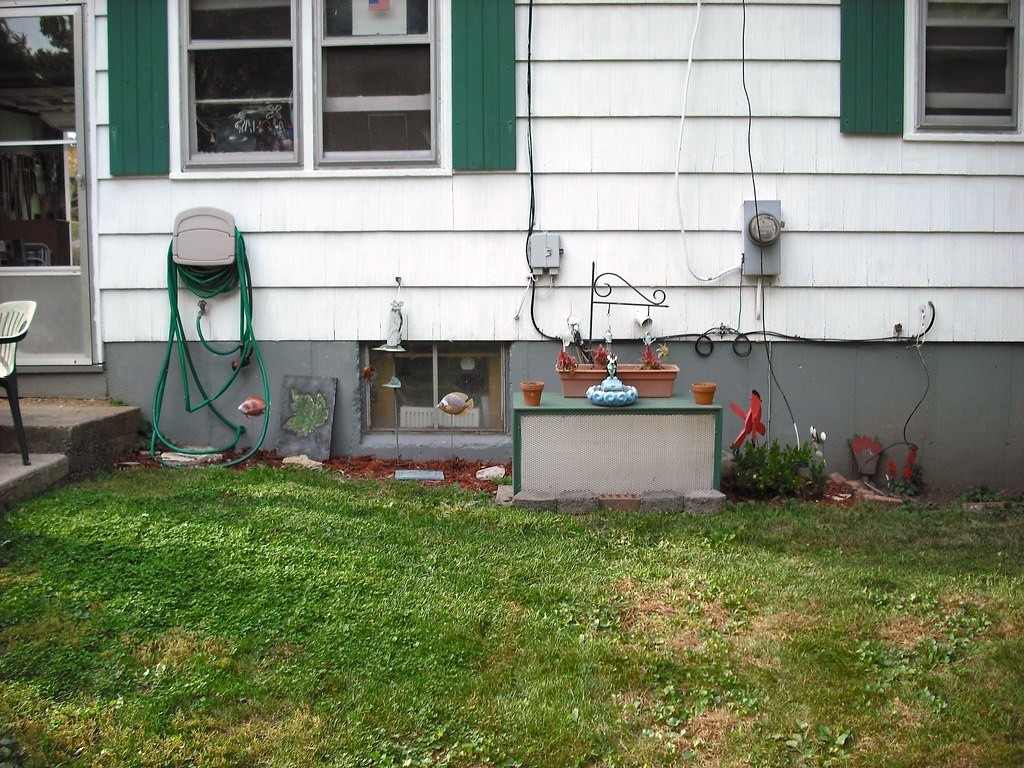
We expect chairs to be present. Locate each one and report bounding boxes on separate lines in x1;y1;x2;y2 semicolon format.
0;299;36;465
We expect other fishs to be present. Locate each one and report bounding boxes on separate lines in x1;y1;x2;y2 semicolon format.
236;395;271;420
435;392;475;416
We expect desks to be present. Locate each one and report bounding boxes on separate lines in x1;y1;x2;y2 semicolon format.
0;218;69;265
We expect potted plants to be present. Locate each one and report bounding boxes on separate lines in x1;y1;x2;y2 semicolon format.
554;343;678;398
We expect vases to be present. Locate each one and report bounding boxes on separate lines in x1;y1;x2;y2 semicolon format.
521;381;545;406
692;382;717;406
